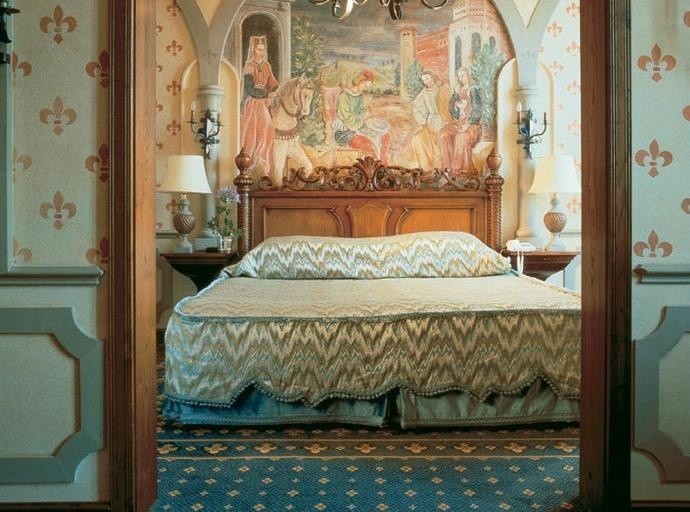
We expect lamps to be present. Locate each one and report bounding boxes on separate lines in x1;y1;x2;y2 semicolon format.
515;99;581;251
156;102;226;254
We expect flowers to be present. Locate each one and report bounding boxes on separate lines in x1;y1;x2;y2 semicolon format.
207;184;241;238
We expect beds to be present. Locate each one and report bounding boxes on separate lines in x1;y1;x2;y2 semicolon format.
162;148;581;427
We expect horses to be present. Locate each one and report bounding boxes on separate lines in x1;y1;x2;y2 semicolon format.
268;72;315;192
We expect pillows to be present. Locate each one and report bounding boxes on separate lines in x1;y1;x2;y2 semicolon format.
225;230;513;280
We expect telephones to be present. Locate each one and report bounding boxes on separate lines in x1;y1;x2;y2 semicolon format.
505;239;536;253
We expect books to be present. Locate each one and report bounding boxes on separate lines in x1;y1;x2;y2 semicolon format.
536;247;544;252
559;232;581;238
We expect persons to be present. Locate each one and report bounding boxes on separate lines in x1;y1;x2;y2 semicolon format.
438;62;483;174
237;33;279;179
333;68;393;166
412;69;449;173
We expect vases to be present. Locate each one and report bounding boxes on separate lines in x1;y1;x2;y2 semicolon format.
218;231;234;251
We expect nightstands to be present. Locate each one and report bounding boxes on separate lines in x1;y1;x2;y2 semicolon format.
502;245;580;283
158;250;236;292
